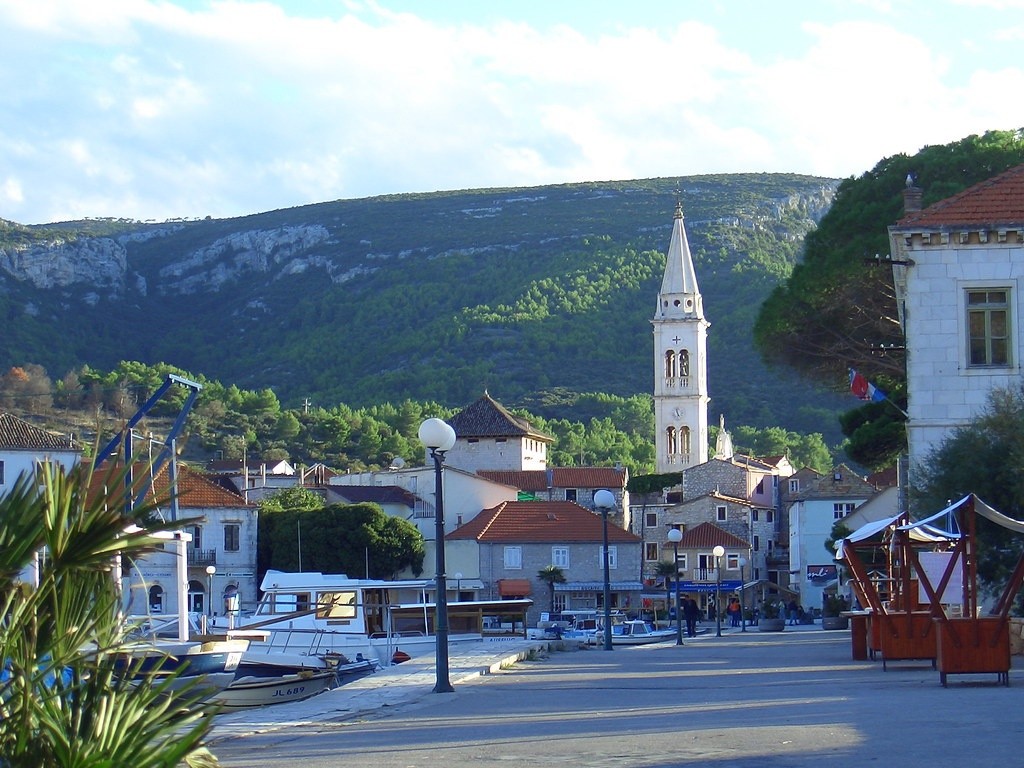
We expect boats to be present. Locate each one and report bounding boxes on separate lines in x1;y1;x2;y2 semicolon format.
530;609;707;645
0;372;532;716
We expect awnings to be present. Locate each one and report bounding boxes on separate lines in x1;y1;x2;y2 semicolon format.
498;579;531;595
553;581;643;590
412;580;484;590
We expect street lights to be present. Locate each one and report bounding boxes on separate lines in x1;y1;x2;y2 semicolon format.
712;547;726;636
205;565;216;615
418;418;457;696
456;572;461;602
738;557;748;632
594;490;615;650
668;529;684;644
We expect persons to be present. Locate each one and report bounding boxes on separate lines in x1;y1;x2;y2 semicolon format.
726;597;742;627
683;595;701;638
789;597;799;626
666;601;676;620
779;599;785;617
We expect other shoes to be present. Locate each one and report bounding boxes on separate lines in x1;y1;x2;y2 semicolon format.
789;624;794;626
732;625;738;627
689;633;697;637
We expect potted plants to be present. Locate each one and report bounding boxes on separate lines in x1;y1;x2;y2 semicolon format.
758;596;785;632
821;597;850;630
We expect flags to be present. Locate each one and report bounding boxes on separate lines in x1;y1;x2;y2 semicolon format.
848;369;885;402
221;588;238;598
